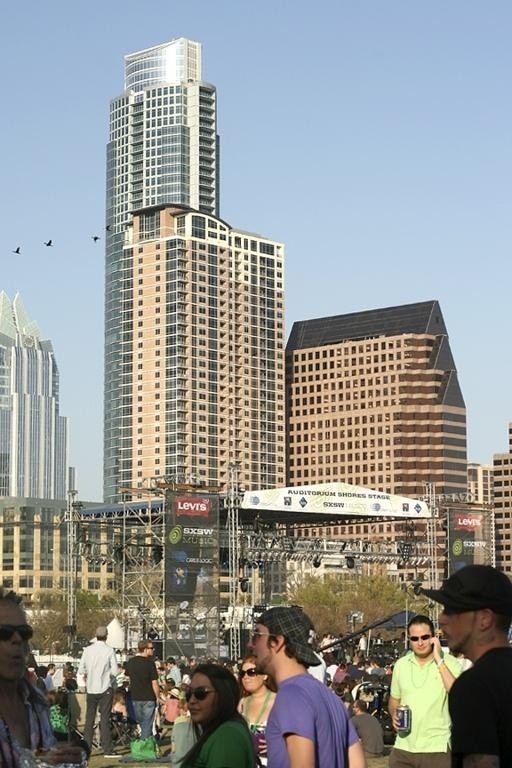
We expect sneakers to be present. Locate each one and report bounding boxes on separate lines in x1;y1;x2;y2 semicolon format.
103;750;125;759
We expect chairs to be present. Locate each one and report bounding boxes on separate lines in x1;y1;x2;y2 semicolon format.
67;692;173;750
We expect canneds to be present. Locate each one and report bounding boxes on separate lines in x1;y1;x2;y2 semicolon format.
395;704;411;733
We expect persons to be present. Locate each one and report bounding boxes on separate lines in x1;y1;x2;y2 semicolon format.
418;562;512;768
0;585;89;768
387;614;461;768
319;630;406;758
246;605;367;767
27;625;277;767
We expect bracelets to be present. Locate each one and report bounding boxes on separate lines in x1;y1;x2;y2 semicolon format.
439;664;447;673
437;658;444;668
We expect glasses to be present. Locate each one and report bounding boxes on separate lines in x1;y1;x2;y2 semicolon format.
184;686;216;702
407;634;434;642
0;624;35;642
252;631;276;640
238;668;257;677
147;647;154;650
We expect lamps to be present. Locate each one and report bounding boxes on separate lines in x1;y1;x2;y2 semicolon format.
78;544;168;569
238;539;433;570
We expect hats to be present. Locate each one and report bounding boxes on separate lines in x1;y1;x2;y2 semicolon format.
420;565;512;611
96;627;109;638
258;606;323;667
